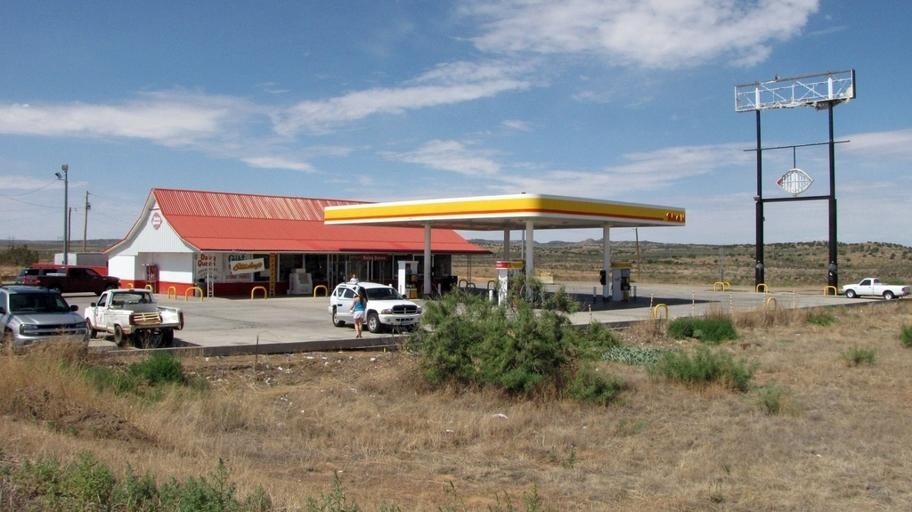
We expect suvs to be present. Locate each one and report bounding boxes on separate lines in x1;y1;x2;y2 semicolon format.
0;283;91;357
326;279;423;335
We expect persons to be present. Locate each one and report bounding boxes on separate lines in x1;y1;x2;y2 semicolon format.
349;272;359;282
349;286;369;338
622;278;630;304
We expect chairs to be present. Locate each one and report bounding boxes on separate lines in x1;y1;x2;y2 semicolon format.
139;299;147;303
116;300;125;304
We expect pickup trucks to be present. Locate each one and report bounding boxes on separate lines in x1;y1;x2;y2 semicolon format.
83;286;184;349
16;262;122;297
840;277;911;301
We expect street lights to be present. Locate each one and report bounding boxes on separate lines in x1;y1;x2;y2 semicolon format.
55;163;70;267
